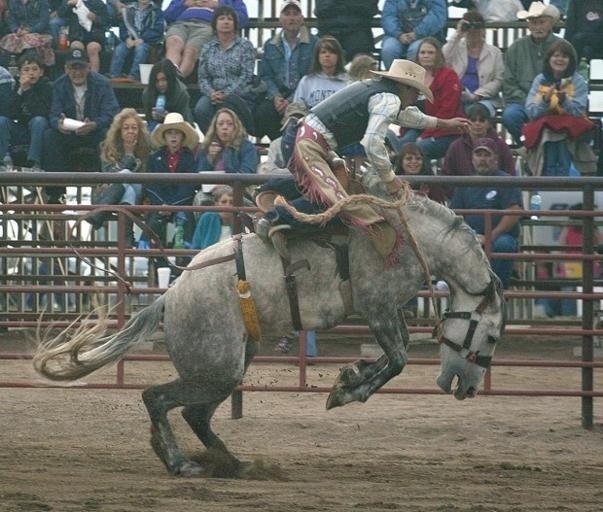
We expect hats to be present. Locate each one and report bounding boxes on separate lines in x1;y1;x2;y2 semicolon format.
64;48;90;66
368;58;436;104
516;1;561;25
150;112;200;151
280;0;302;13
472;137;497;156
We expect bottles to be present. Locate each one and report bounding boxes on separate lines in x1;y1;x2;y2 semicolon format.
157;266;170;289
62;116;83;135
529;190;541;222
577;57;590;83
152;94;169;116
172;223;186;248
58;29;68;49
104;32;117;52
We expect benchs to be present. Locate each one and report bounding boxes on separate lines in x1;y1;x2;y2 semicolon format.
0;0;601;327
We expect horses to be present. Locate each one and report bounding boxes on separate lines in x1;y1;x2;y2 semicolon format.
24;166;508;479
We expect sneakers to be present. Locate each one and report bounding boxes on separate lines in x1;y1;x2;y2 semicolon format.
263;213;293;263
274;335;295;354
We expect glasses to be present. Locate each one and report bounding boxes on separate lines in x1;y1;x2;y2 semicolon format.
470;118;488;124
